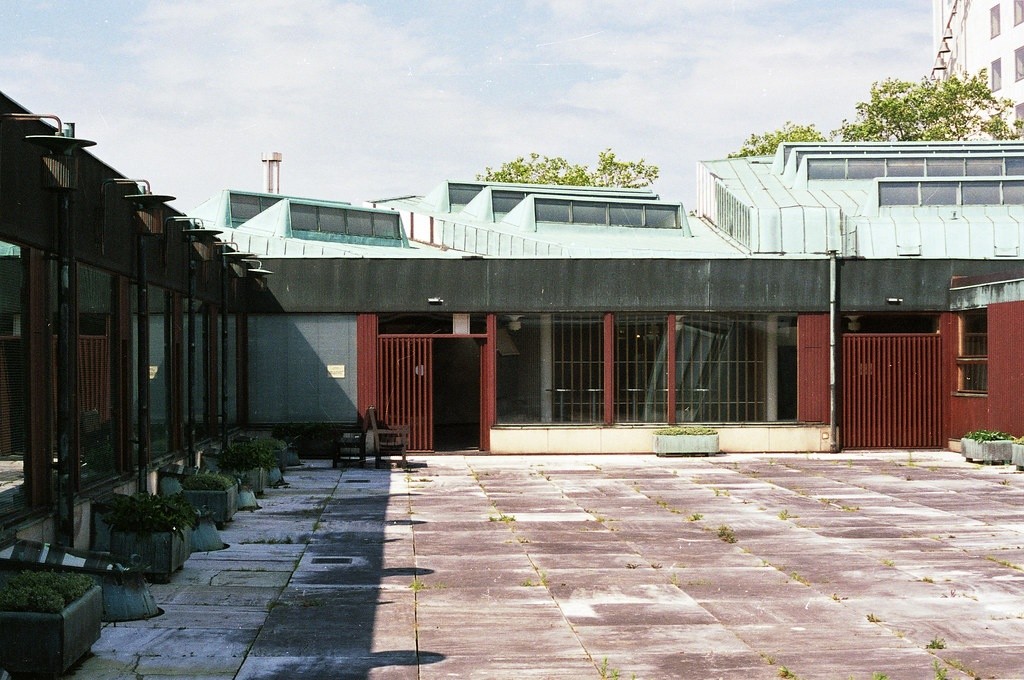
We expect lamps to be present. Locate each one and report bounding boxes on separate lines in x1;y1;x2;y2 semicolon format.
99;178;275;277
1;113;98;154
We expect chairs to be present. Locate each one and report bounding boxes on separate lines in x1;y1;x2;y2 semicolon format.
369;406;408;468
332;407;370;469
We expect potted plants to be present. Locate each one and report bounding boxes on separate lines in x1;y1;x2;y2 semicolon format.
0;572;103;676
181;436;289;529
652;427;719;457
960;429;1018;465
99;491;199;584
1012;435;1024;471
